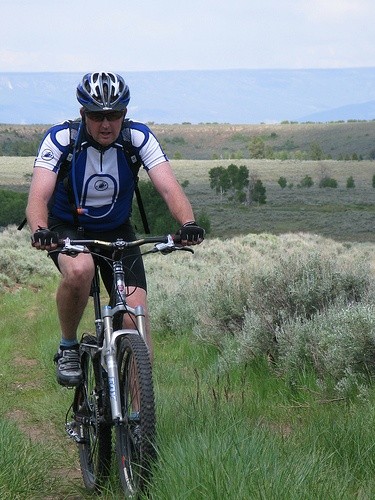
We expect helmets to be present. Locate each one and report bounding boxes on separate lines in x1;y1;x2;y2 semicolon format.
75;71;130;112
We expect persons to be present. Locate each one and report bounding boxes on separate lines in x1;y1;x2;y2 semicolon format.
25;72;207;463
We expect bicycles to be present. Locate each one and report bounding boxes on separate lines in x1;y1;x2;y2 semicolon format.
29;228;199;499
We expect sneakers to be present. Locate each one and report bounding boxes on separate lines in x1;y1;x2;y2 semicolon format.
128;417;144;461
53;342;84;387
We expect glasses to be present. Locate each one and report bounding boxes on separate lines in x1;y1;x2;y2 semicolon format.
85;110;124;121
